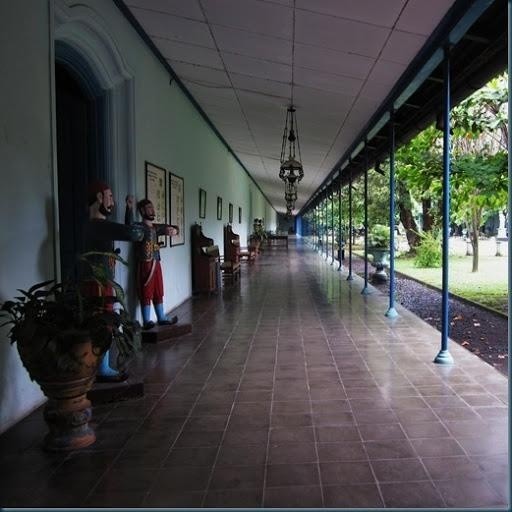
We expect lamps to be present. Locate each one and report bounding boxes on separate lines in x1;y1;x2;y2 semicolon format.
279;105;304;222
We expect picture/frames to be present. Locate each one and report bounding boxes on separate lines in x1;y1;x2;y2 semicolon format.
169;172;185;247
239;208;242;223
229;203;233;223
199;188;206;218
217;197;222;220
146;161;166;248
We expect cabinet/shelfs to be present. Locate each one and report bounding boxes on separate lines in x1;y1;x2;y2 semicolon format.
225;224;240;263
191;222;220;295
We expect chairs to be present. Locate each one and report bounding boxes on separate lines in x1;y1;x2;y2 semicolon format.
215;246;241;286
240;246;256;265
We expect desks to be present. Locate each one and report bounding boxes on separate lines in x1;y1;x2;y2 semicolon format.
269;236;288;250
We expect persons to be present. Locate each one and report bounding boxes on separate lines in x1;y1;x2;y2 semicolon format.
124;195;180;329
78;180;143;382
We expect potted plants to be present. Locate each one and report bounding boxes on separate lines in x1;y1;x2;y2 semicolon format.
251;231;260;255
315;224;327;246
367;224;391;284
0;251;141;451
335;229;348;259
